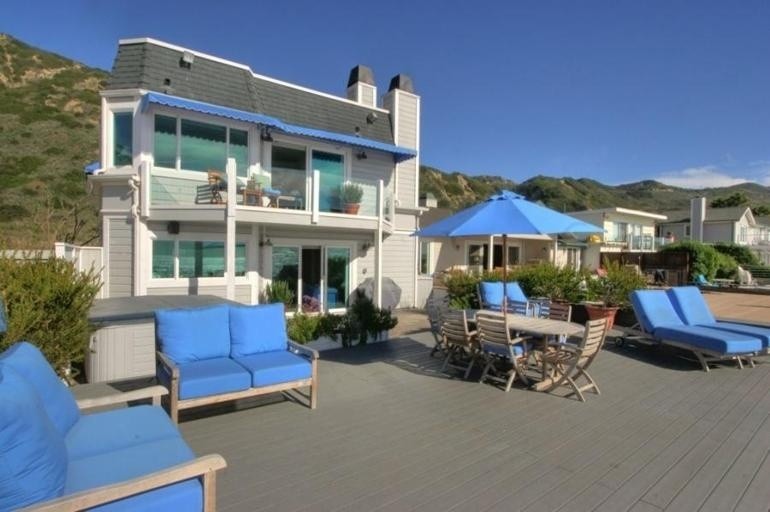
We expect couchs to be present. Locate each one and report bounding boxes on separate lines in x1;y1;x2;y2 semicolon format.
154;303;319;425
1;339;227;511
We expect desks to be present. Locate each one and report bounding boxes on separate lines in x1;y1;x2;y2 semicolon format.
275;193;303;210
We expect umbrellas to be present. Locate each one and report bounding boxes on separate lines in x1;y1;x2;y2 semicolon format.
409;189;609;317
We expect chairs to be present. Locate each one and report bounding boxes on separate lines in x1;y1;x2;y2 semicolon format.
427;281;608;402
616;285;770;372
208;169;286;207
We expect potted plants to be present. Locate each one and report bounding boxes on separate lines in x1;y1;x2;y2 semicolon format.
288;286;399;352
584;255;623;330
336;182;366;214
